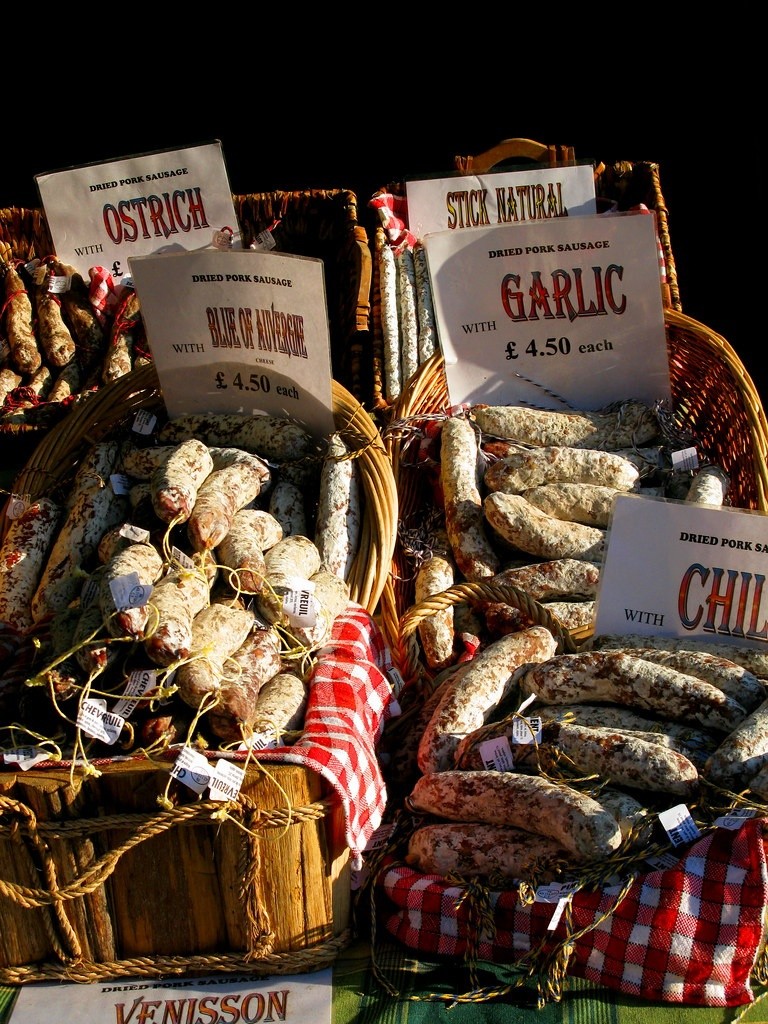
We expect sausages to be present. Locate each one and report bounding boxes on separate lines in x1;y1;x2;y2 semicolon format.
0;251;360;750
404;406;768;884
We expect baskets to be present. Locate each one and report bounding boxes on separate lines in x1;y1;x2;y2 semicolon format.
0;361;397;750
387;305;768;681
0;189;376;404
362;620;768;990
371;137;683;408
